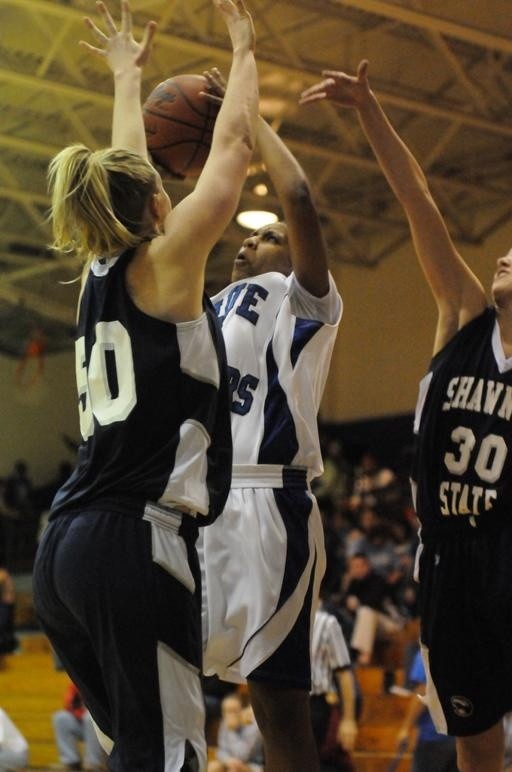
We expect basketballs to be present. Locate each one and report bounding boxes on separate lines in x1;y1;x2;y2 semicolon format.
142;74;222;176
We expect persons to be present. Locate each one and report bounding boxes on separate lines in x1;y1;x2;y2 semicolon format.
185;67;343;769
33;3;261;771
0;432;458;771
299;59;511;772
